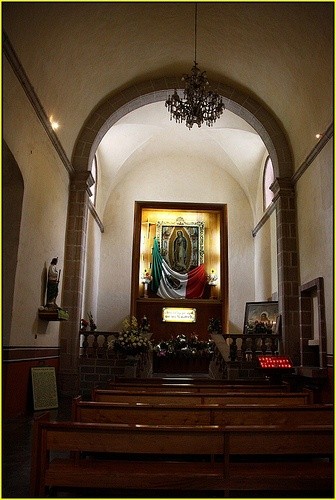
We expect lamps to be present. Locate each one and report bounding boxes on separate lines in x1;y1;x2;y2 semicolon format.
165;3;227;131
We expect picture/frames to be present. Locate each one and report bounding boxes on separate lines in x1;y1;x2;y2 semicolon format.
240;301;282;354
155;216;205;275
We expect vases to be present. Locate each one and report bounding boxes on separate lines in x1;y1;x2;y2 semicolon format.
82;326;87;331
90;327;95;331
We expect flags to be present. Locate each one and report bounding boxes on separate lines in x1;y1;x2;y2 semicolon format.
151;238;206;299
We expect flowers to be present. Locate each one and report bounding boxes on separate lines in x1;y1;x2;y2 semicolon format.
80;319;88;328
107;313;239;366
87;310;97;329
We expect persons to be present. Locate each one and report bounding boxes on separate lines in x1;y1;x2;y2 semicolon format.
46;256;62;306
173;230;187;271
86;310;97;331
208;269;218;285
254;311;270;333
141;269;150;284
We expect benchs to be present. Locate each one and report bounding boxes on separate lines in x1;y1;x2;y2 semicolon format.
32;372;334;499
